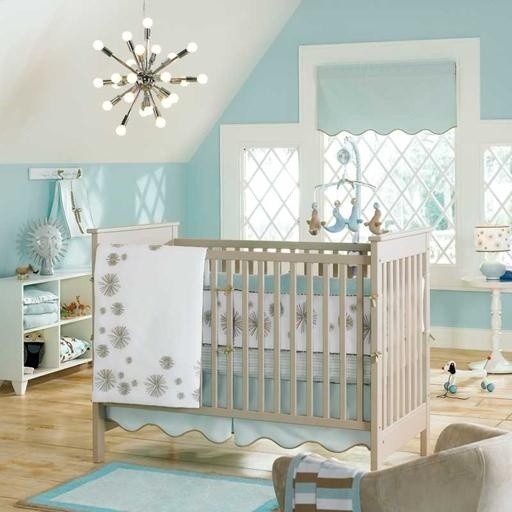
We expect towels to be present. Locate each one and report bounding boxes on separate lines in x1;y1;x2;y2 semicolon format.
281;452;368;512
92;243;207;408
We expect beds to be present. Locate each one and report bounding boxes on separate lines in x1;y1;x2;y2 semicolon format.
87;222;432;477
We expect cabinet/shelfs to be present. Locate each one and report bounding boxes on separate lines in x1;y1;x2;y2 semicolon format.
0;269;91;396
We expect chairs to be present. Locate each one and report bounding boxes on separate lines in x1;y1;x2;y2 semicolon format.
272;421;512;512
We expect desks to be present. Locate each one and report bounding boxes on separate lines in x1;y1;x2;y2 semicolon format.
462;275;511;374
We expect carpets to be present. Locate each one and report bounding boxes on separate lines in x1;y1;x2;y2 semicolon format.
15;460;278;512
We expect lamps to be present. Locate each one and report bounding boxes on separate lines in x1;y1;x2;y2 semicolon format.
473;223;511;279
90;18;208;138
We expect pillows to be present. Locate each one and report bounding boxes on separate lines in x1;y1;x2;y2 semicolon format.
59;335;90;363
